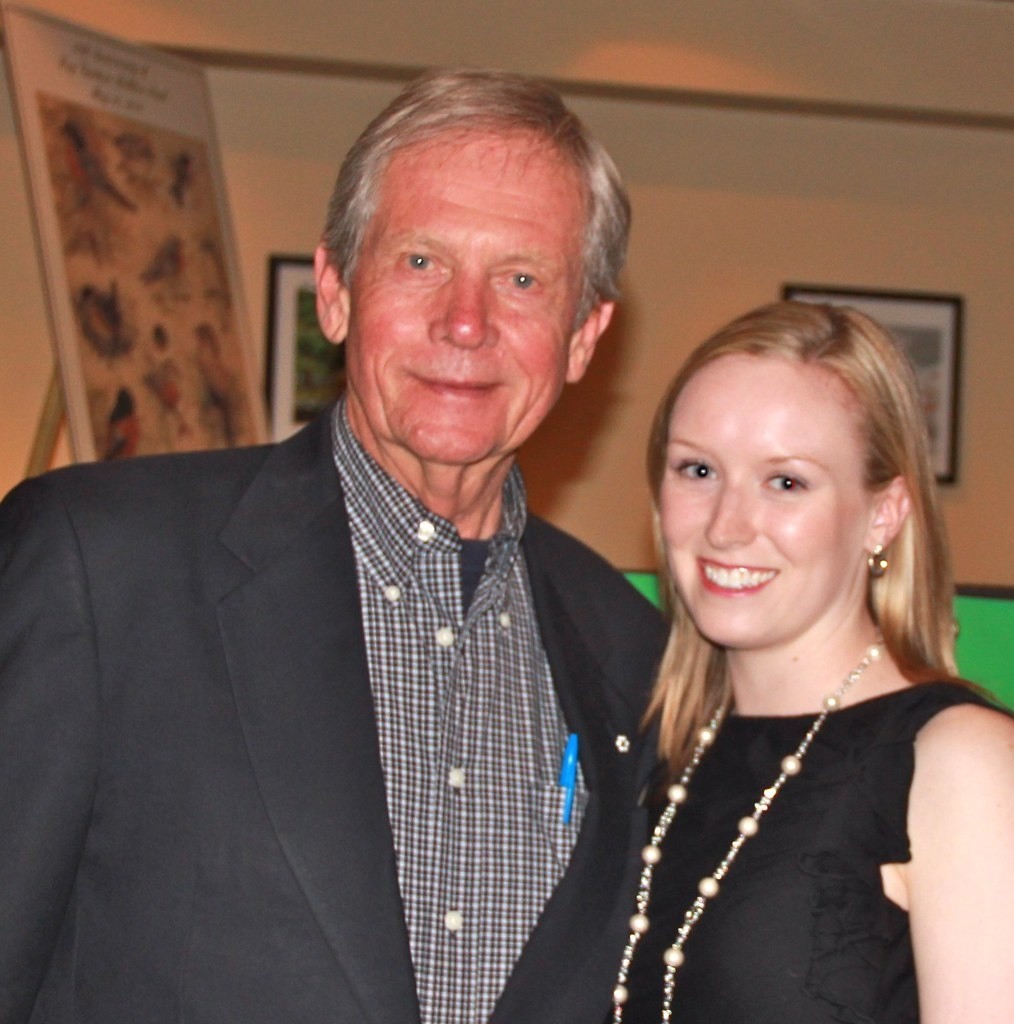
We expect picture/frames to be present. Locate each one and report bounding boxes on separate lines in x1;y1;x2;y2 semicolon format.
265;254;346;444
779;280;965;486
0;5;266;466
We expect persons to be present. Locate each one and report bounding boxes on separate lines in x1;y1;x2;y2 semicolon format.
0;71;666;1021
606;300;1014;1024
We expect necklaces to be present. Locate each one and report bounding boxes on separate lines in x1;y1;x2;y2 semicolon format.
614;639;884;1024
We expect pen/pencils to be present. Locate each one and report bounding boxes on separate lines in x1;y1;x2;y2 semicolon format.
559;732;579;825
